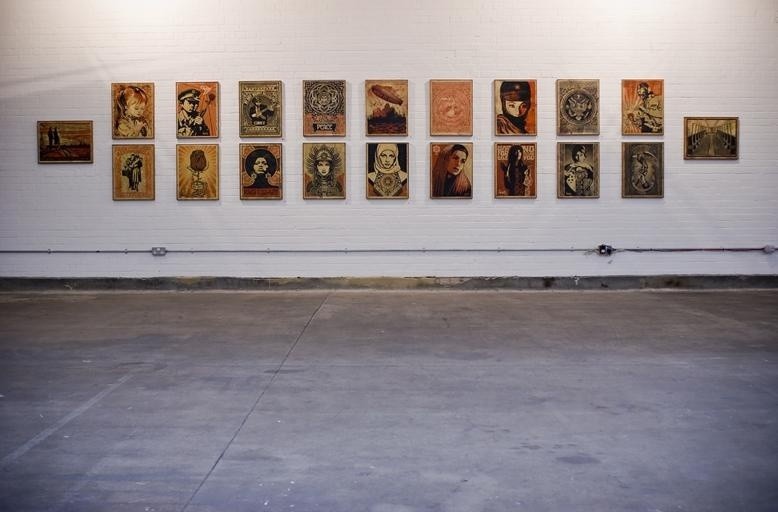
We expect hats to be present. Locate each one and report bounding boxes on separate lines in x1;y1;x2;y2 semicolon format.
178;89;200;102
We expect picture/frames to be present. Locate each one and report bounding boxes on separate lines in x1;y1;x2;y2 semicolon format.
554;144;598;198
494;78;538;137
238;142;284;201
111;143;157;201
238;80;283;139
682;116;738;159
429;141;472;200
301;141;347;200
429;78;472;138
302;79;348;138
554;79;598;134
493;141;538;200
110;82;156;140
363;79;410;138
37;121;93;164
365;141;410;200
174;143;221;201
620;142;662;198
175;81;220;139
620;78;664;136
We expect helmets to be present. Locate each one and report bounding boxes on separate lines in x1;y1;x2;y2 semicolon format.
500;81;531;101
